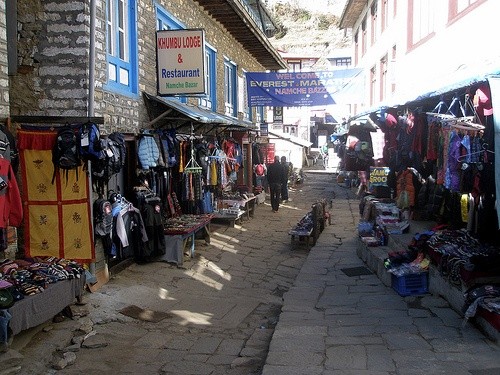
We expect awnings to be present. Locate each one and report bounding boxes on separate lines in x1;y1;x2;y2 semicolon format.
142;91;259;136
341;41;500;132
268;130;315;148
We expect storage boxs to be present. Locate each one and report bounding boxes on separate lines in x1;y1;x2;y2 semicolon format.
390;271;429;297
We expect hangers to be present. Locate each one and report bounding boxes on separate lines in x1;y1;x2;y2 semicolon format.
134;183;161;203
110;190;133;210
457;143;495;163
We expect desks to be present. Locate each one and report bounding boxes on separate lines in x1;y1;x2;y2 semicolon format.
151;214;216;266
216;210;246;229
288;215;313;253
215;196;256;220
256;190;267;205
6;260;86;335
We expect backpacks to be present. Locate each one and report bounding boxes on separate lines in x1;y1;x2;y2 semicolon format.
92;129;178;197
92;193;113;238
51;122;82;185
76;121;101;176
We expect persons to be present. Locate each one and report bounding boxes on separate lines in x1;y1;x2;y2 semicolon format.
280;156;289;203
267;156;287;213
